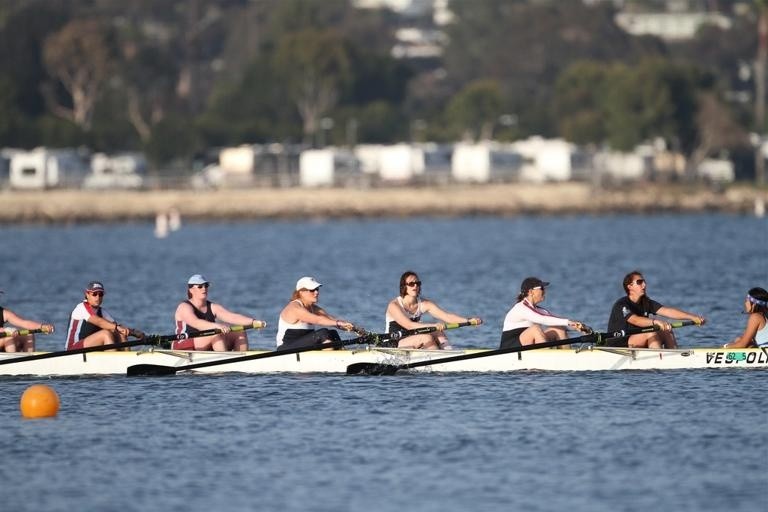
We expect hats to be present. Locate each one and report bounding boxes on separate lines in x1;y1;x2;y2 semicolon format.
522;278;550;291
188;275;210;285
296;277;323;292
86;282;104;291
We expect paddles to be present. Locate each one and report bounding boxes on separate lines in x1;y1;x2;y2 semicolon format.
127;317;477;377
347;321;700;377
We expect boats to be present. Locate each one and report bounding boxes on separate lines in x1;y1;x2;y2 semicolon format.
0;346;768;377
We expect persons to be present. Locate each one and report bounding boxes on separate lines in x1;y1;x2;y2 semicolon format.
715;287;768;348
0;290;54;352
64;281;146;351
276;277;355;350
170;275;267;352
499;278;583;349
383;272;481;350
607;272;705;349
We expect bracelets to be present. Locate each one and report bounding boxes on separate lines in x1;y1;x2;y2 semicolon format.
336;319;340;327
41;323;45;331
251;318;256;326
652;319;656;326
464;317;471;323
115;324;120;333
722;344;727;348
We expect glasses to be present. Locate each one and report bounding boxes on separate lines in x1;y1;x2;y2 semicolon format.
532;287;544;290
301;288;319;292
193;284;210;288
405;280;421;287
630;279;646;284
89;292;104;297
